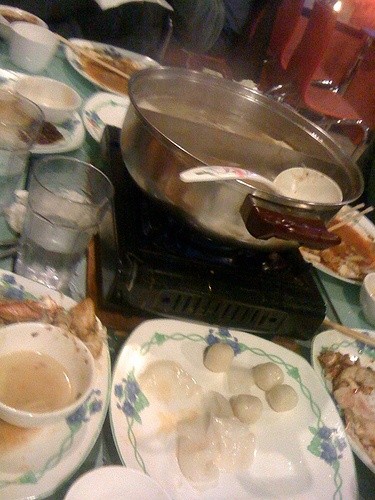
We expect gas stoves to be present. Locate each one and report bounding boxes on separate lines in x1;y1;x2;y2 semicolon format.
89;123;327;343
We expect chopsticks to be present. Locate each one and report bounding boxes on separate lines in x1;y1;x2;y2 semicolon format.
54;34;132;81
327;203;374;231
0;238;19;260
323;319;375;348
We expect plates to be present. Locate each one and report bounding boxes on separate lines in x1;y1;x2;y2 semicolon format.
62;464;169;500
0;67;86;155
65;37;166;98
310;328;375;475
0;269;112;499
107;319;361;500
298;204;375;285
82;90;130;142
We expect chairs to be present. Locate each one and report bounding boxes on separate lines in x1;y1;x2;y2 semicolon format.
259;0;334;94
276;0;369;161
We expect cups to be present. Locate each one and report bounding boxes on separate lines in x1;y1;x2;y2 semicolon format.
0;88;47;258
8;21;62;74
14;155;116;295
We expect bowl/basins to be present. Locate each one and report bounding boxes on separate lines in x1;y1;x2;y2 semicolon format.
1;319;95;431
359;273;375;327
0;4;49;46
16;76;82;124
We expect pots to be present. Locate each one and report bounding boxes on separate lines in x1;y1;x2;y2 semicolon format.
118;51;364;249
